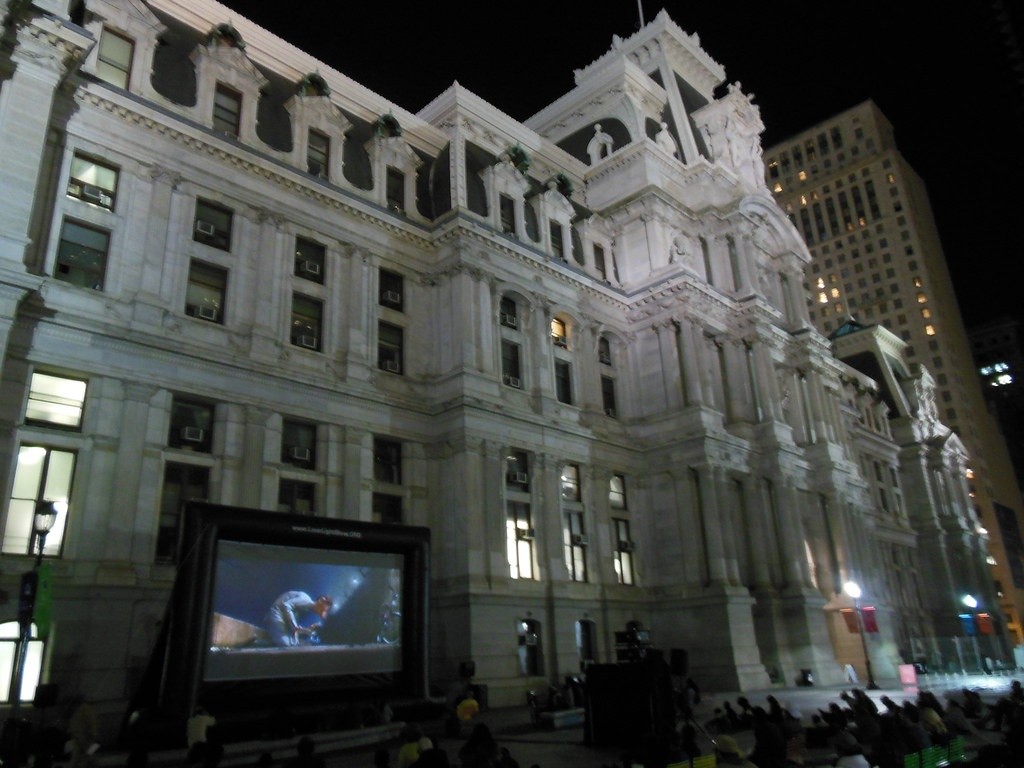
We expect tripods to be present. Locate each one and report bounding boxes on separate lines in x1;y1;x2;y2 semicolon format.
676;677;717;746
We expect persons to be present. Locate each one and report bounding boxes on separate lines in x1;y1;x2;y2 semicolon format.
269;590;334;645
244;736;328;768
62;695;107;768
373;688;450;768
621;700;808;768
459;724;520;768
183;706;225;741
456;687;484;722
811;680;1024;768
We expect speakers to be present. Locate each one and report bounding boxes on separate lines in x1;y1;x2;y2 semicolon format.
34;684;59;708
460;660;475;678
671;649;689;677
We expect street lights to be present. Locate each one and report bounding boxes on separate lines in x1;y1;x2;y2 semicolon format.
13;502;57;709
843;580;879;690
963;593;987;672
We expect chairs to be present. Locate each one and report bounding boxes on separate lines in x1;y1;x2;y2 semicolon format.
904;735;969;768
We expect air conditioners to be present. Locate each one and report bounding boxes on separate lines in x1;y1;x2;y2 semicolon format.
300;261;320;276
580;659;593;672
193;305;216;321
621;541;635;551
501;313;516;327
382;290;400;305
507;233;520;241
195;220;216;240
316;173;330;182
510;472;526;484
575;535;589;545
180;426;203;442
506;376;520;388
520;528;536;540
80;185;102;204
604;408;616;418
296;335;316;349
288;447;309;460
517;633;538;646
599;352;610;364
224;131;239;142
558;257;569;264
382;360;399;373
554;335;568;347
